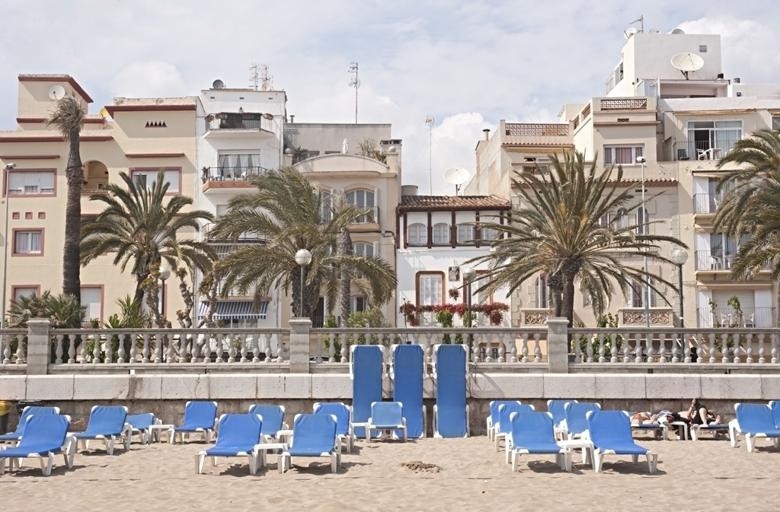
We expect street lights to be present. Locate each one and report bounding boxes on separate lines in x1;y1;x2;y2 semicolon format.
670;246;689;364
0;162;26;332
462;265;476;360
295;248;312;318
637;155;652;327
156;265;170;363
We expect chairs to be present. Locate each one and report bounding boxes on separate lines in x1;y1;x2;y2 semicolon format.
0;345;780;476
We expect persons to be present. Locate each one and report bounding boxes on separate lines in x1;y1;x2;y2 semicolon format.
633;398;721;427
